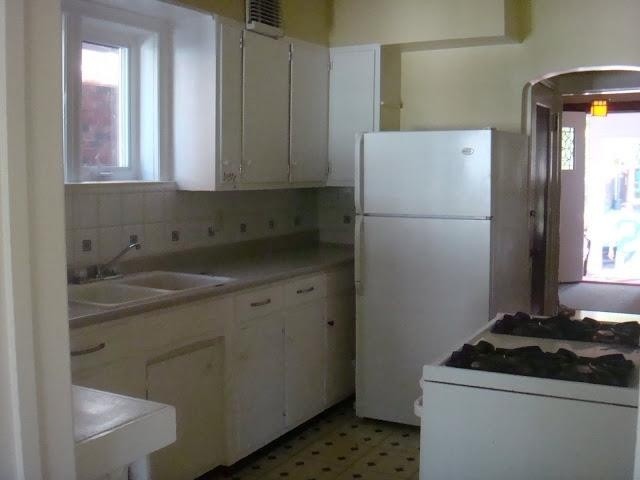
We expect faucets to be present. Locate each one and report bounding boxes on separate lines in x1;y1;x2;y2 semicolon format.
95;243;141;279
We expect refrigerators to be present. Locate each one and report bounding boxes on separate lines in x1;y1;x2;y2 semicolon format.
348;127;531;430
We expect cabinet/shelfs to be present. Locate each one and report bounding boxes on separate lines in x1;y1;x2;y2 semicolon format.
325;262;355;408
239;27;328;183
69;296;223;480
171;18;240;191
330;45;403;187
285;268;327;431
223;279;285;467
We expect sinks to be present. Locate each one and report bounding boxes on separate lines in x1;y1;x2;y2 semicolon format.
117;270;237;291
69;280;166;307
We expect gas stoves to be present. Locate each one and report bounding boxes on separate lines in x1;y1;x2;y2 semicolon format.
434;310;640;391
